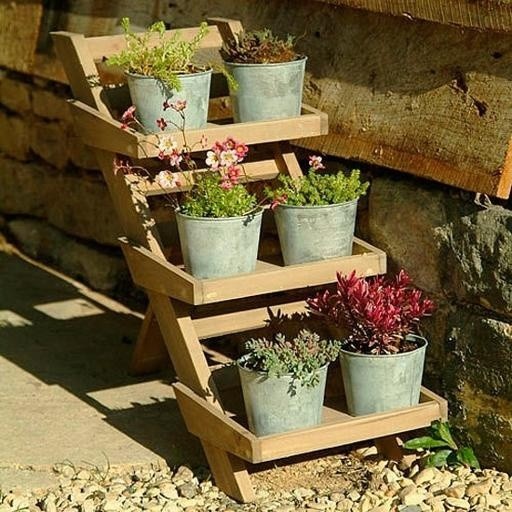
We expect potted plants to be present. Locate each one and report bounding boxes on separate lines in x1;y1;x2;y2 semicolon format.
237;329;341;437
306;270;435;418
218;26;308;123
102;16;238;135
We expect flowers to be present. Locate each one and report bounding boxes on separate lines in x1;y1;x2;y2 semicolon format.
269;153;371;207
112;98;285;216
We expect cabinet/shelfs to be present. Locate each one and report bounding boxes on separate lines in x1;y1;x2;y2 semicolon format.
50;17;449;501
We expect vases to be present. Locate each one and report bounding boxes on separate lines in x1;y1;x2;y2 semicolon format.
272;197;361;266
175;206;265;279
